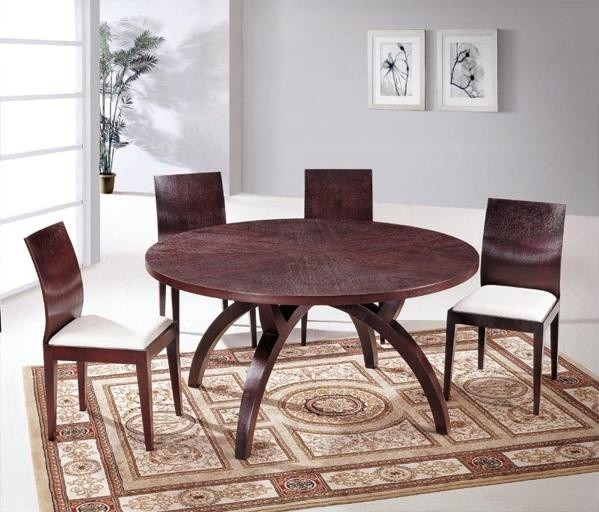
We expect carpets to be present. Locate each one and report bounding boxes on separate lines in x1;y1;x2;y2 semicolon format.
23;327;599;512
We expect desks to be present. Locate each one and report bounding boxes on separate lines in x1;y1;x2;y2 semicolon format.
146;219;479;459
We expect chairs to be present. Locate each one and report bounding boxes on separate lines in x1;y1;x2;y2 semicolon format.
301;169;385;346
444;198;566;415
24;222;182;451
154;172;258;349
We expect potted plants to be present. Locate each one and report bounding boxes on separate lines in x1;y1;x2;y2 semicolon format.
98;22;166;193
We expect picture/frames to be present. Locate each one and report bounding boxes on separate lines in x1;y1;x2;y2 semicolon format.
436;30;497;111
367;30;425;111
